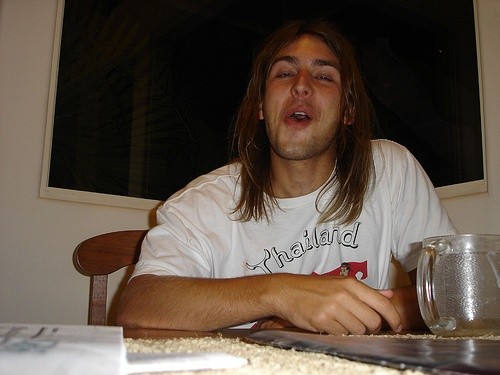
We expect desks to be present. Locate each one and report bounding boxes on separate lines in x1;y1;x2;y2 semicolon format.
0;325;500;375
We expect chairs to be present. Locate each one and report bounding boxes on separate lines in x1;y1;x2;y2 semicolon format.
72;228;149;327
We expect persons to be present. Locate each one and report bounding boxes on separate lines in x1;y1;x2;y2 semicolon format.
116;16;465;339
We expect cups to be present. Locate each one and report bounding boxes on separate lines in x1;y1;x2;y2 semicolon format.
417;232;500;339
